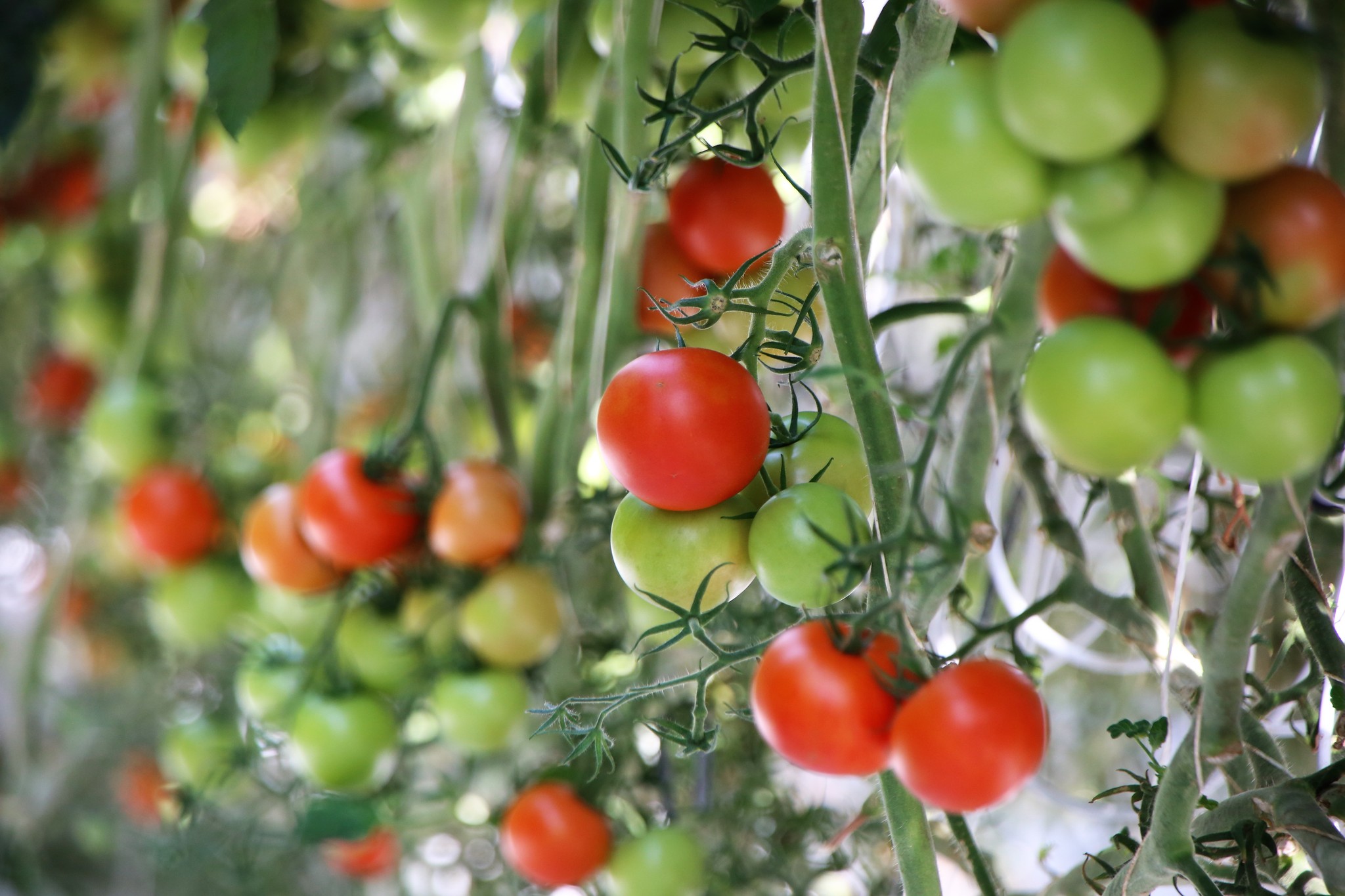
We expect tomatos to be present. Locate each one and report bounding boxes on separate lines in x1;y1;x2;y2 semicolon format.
0;0;1345;896
